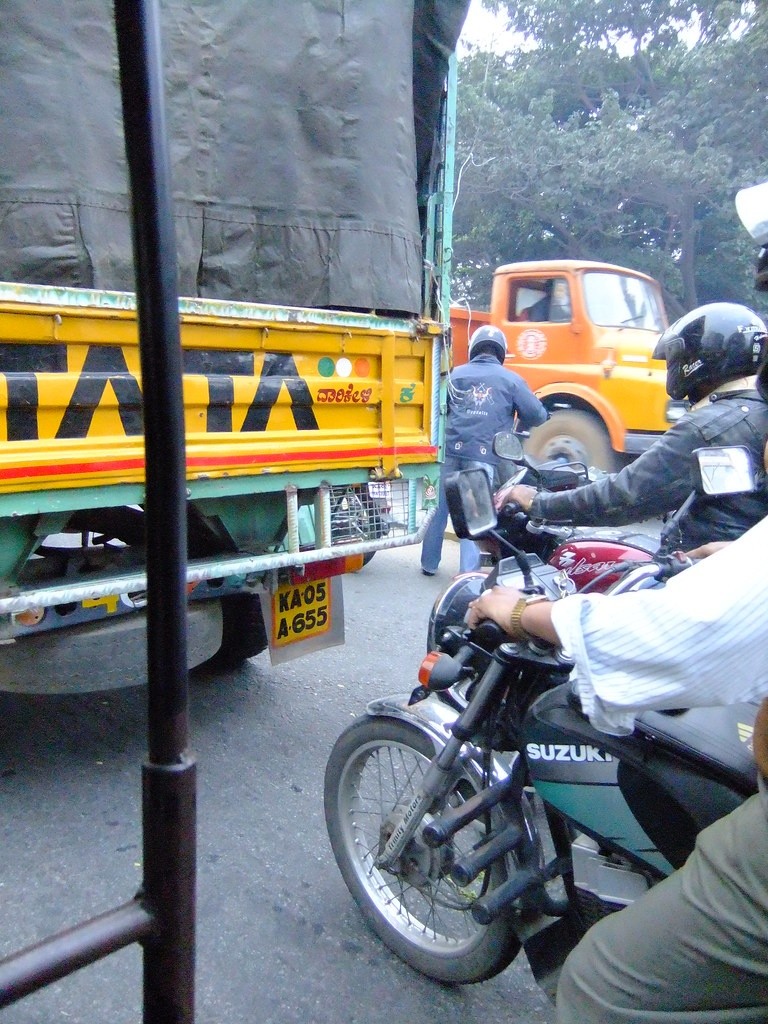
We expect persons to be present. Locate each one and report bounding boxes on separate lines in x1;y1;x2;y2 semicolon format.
493;302;768;560
421;325;551;575
529;279;571;323
465;180;768;1024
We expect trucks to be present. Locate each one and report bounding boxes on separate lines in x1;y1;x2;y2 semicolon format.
1;0;472;690
441;258;687;477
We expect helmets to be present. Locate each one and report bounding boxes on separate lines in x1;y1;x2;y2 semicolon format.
468;325;508;365
731;182;768;278
649;301;766;404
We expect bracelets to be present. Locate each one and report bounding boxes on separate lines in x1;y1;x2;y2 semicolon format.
528;497;534;508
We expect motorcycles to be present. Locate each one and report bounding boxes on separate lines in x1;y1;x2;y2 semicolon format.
330;486;394;566
323;432;757;1019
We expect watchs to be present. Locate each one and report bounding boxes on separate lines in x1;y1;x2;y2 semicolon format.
512;594;549;641
